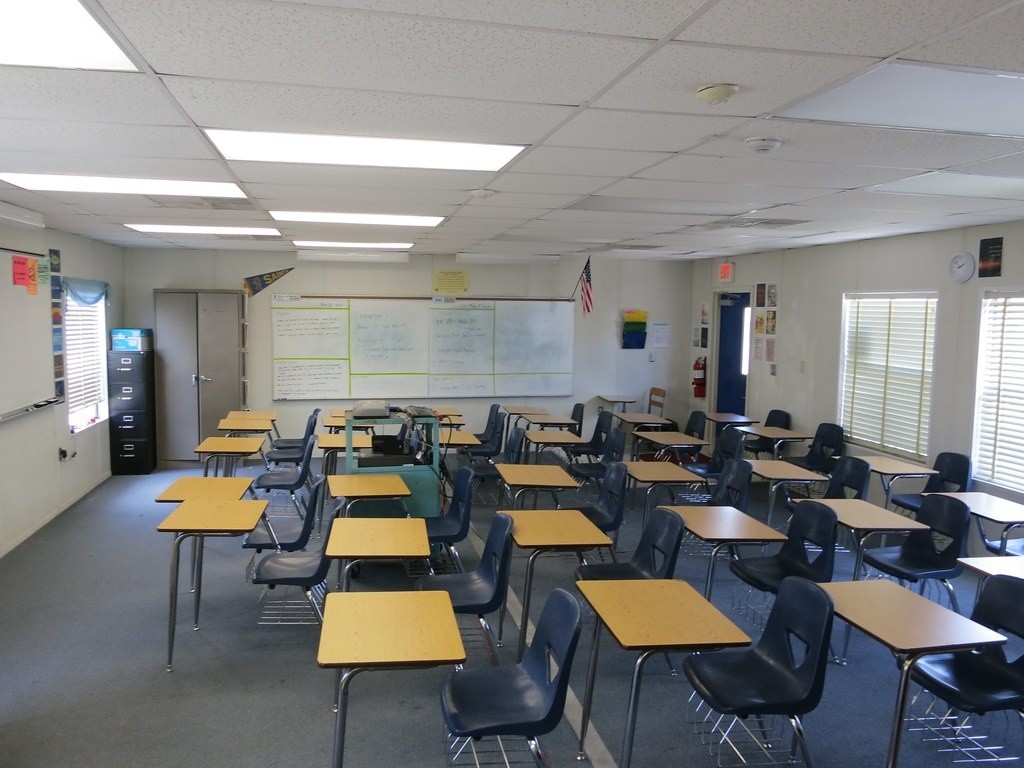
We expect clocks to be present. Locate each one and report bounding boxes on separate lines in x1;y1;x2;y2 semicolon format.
949;252;976;284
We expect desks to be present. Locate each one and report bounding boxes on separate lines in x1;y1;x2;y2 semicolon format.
632;432;710;466
621;462;707;533
502;406;553;452
218;419;273;477
744;460;829;558
599;395;637;413
327;475;411;589
514;414;579;446
226;411;280;477
921;492;1024;557
734;426;815;498
325;517;434;592
832;455;940;579
421;430;481;449
432;408;463;417
792;499;930;666
706;413;760;442
497;510;617;664
956;556;1024;608
438;416;465;431
157;477;259;592
194;436;269;477
156;499;282;673
316;591;468;768
575;578;752;768
614;413;672;462
525;431;586;465
815;578;1008;768
318;408;376;537
656;505;788;601
495;464;580;510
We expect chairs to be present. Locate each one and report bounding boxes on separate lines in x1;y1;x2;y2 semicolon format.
242;474;329;596
395;385;844;505
897;574;1024;768
252;496;347;626
576;507;685;676
414;512;514;667
440;588;583;768
706;458;753;560
254;435;319;521
860;494;971;614
422;467;476;572
273;408;322;449
265;414;318;492
987;538;1024;556
891;452;972;521
578;462;627;563
729;500;840;664
683;576;835;768
822;457;873;500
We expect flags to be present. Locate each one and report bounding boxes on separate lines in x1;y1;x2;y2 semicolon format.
579;258;593;319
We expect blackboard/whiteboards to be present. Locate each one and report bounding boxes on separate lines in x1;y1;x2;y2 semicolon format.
270;293;575;401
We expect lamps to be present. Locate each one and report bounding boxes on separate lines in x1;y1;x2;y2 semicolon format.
455;253;561;264
297;250;409;262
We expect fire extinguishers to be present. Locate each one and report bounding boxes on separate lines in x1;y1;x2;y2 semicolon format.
693;358;707;399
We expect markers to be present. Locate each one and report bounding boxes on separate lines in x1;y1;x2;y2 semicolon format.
275;398;287;400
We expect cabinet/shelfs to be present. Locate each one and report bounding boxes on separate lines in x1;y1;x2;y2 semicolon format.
153;288;246;471
345;411;440;517
108;351;157;475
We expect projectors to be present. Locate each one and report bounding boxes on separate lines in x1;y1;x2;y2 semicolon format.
353;399;389;420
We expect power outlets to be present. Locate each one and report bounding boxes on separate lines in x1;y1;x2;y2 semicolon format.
60;447;67;462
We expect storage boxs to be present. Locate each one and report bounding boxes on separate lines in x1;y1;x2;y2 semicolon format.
111;328;151;351
